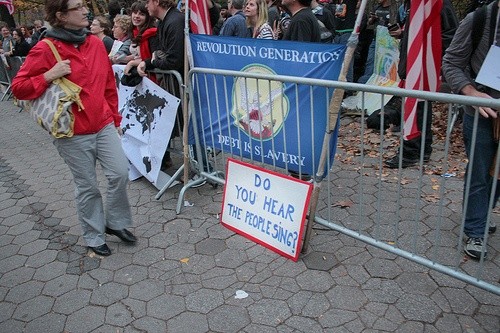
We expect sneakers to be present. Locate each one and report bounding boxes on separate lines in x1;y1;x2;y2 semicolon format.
463;233;487;259
487;220;496;234
180;167;212;188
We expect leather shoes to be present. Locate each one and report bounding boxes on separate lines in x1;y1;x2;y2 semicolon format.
105;227;138;242
423;155;430;162
91;243;111;255
384;156;419;168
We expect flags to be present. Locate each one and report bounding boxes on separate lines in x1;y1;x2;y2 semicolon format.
403;0;443;140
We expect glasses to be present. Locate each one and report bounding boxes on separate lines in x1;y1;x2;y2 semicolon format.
63;3;89;12
113;25;120;29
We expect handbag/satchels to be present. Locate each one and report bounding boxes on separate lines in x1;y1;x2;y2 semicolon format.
15;38;85;139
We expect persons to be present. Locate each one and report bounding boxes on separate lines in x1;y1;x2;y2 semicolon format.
0;0;216;188
207;0;433;168
13;0;138;255
443;0;500;258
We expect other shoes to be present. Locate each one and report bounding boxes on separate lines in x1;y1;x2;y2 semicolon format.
161;159;172;170
